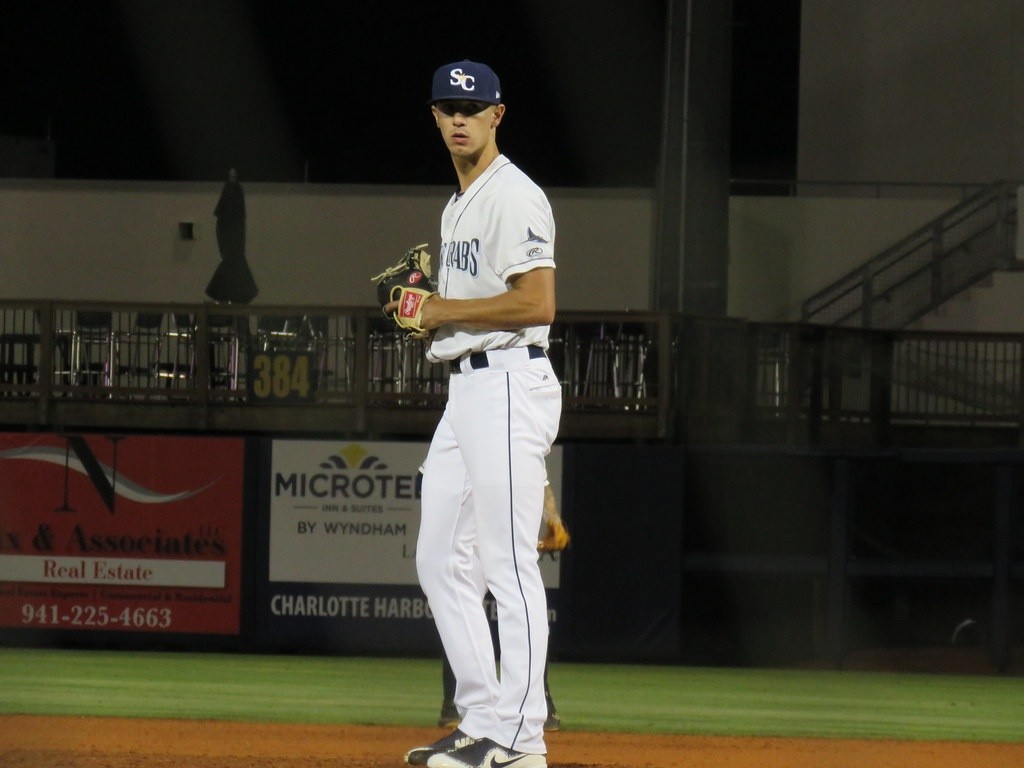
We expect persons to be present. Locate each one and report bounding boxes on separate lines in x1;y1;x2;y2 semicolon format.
376;58;572;768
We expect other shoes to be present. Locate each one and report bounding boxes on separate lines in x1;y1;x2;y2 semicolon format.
438;707;461;727
543;705;561;731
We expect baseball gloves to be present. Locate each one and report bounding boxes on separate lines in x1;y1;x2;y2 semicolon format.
371;242;439;343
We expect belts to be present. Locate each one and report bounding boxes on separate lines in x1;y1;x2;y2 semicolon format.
449;344;545;374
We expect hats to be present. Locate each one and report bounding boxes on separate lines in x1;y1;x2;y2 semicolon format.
431;60;500;105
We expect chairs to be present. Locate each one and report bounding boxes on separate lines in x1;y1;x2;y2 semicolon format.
57;311;652;416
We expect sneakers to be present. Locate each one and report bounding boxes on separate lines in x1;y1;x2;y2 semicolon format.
404;729;548;768
